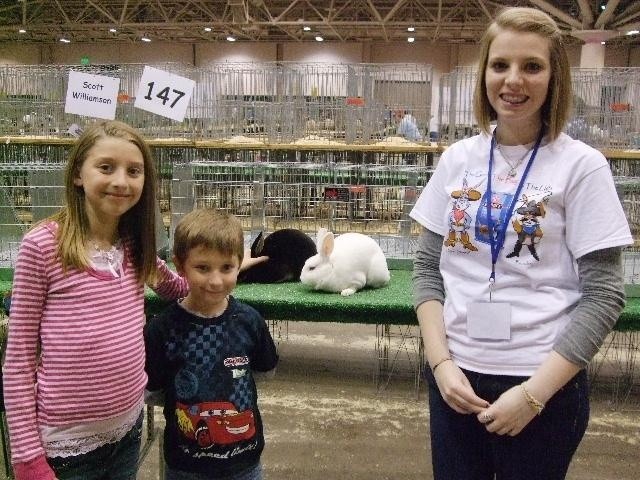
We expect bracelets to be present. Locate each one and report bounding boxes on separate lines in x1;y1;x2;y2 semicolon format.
518;382;545;415
429;357;453;374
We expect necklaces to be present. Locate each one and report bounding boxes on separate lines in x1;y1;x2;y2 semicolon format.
490;126;534;179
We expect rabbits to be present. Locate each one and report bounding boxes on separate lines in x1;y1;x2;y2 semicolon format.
299;227;391;298
236;228;318;285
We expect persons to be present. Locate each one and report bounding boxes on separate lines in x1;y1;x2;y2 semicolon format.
3;119;269;478
394;104;420;140
408;6;632;480
138;208;280;480
426;114;439;141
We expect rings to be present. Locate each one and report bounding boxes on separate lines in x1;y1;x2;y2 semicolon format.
483;412;492;425
